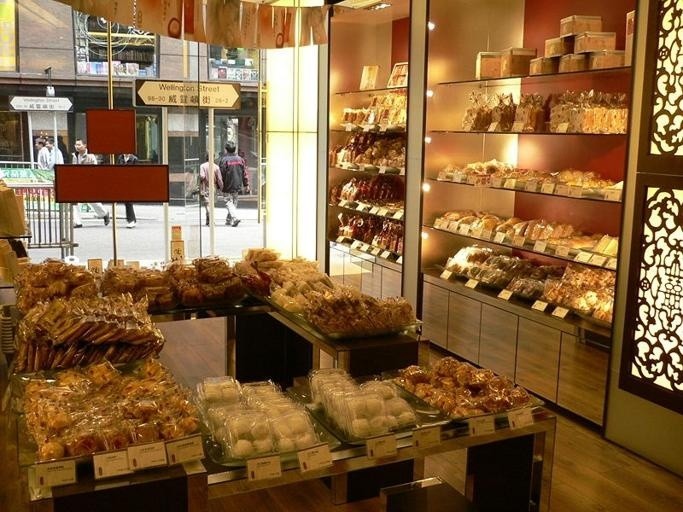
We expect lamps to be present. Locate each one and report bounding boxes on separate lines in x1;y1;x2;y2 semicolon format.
45;86;56;97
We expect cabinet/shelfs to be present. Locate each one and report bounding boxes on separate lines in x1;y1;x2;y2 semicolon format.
417;0;640;433
323;0;413;303
14;294;556;512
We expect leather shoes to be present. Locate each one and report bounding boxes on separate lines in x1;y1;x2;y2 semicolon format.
74;224;82;228
103;211;110;226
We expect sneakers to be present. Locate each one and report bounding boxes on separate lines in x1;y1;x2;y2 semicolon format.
127;221;136;227
232;218;240;226
225;218;231;225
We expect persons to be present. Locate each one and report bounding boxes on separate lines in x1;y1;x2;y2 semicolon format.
114;152;139;228
45;137;64;171
218;141;251;228
198;153;224;227
68;138;111;229
34;138;49;170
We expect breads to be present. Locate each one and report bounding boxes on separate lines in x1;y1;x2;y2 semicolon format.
445;246;616;323
437;158;623;202
397;355;530;419
308;286;415;338
14;255;244;315
309;366;415;440
22;358;199;461
339;95;407;129
233;246;334;312
460;89;628;134
433;208;619;257
17;294;166;371
331;174;404;215
336;211;404;253
195;374;319;462
330;132;406;167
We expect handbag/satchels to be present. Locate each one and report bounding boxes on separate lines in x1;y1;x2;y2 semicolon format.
198;178;209;206
